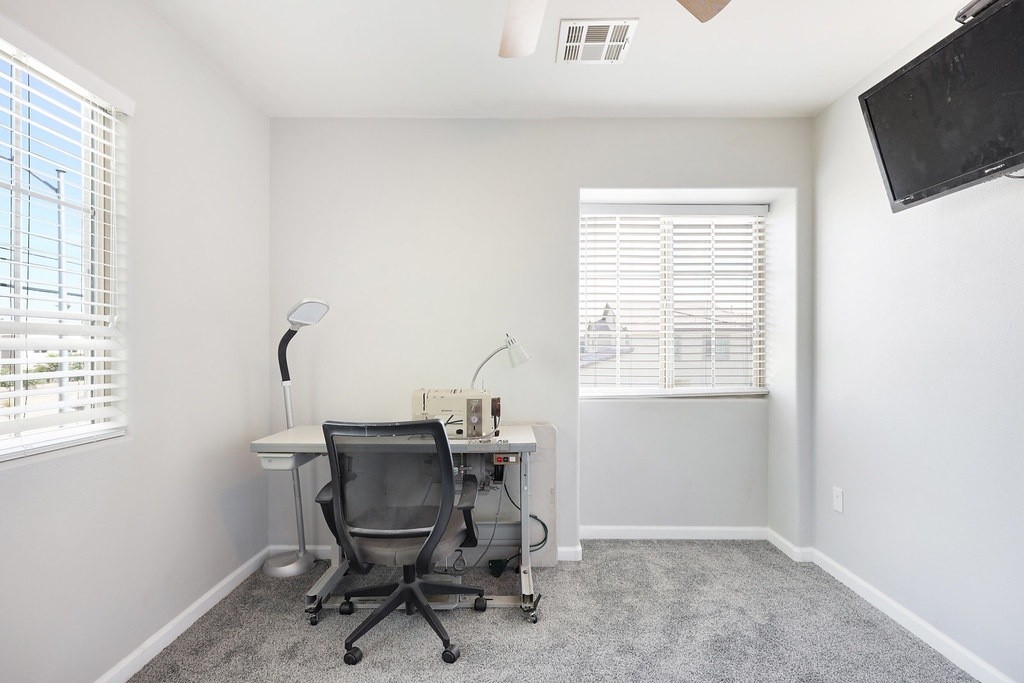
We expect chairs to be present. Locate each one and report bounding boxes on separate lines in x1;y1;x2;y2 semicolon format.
304;420;488;665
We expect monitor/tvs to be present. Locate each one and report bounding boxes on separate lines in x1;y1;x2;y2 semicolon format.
858;0;1023;214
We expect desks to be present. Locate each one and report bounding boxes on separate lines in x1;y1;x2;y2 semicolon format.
255;421;543;622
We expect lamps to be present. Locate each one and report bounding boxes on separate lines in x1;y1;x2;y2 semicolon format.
262;300;330;578
471;333;528;389
